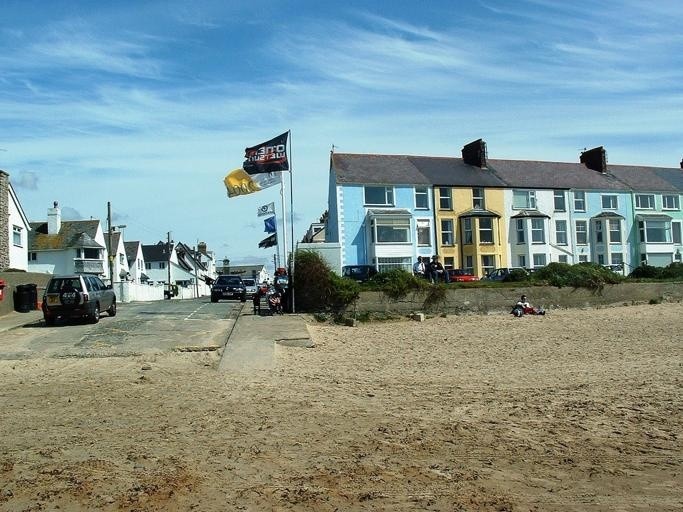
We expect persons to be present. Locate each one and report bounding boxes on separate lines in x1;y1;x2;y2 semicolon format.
412;256;432;282
516;295;545;316
268;293;283;311
251;290;262;315
276;270;291;314
429;255;452;284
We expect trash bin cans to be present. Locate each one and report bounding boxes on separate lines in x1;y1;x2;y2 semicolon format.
15;284;37;312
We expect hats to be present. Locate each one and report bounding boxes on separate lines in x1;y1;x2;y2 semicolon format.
431;255;438;259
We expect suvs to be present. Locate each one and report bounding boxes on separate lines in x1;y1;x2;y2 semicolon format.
42;273;117;325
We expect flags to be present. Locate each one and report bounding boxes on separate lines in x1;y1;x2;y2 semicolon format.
257;202;274;217
243;131;289;175
223;168;282;198
258;234;278;249
264;216;276;234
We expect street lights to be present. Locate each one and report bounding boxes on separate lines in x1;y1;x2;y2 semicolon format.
107;201;126;286
167;232;175;300
193;245;202;298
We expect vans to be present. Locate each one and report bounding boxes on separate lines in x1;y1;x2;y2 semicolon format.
342;265;378;281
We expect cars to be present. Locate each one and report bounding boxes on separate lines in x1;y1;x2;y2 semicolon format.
444;268;479;282
602;264;624;277
205;273;269;303
481;266;530;281
